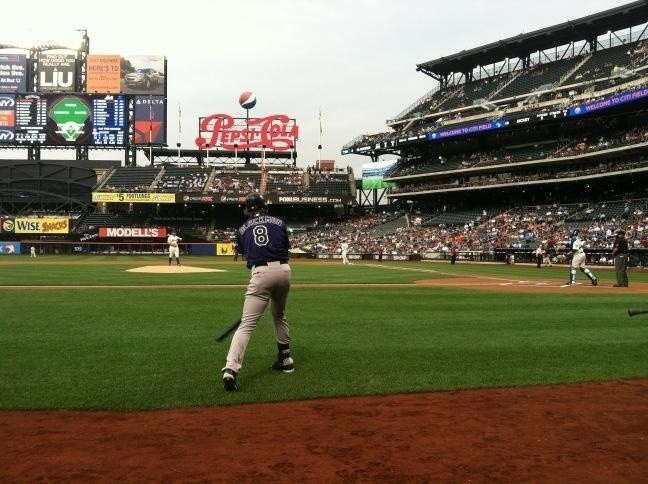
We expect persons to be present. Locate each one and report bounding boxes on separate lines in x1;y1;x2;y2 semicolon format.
612;230;629;287
99;162;334;266
219;193;295;392
351;43;648;149
296;118;648;268
30;245;36;258
565;236;598;287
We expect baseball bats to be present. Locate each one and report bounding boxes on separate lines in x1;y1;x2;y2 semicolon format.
215;319;241;342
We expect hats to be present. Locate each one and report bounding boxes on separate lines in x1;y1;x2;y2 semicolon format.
243;193;265;210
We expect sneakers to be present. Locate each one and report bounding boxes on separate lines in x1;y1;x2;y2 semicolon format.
592;278;597;286
613;284;628;287
222;368;238;392
566;282;576;285
272;357;294;373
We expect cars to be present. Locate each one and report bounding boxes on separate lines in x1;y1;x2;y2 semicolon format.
125;68;159;89
